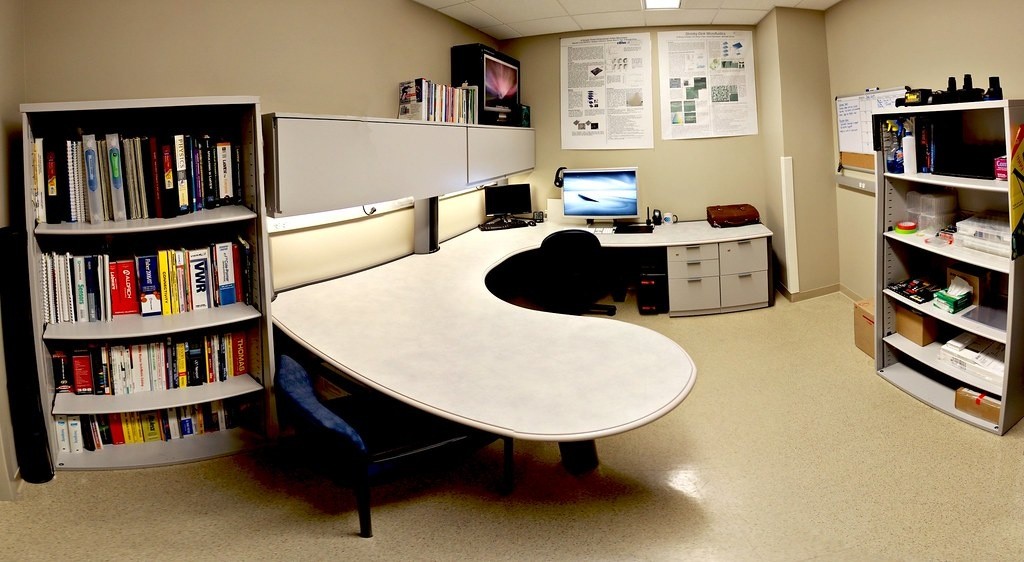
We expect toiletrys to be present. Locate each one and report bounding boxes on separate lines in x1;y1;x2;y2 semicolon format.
902;121;917;175
886;120;904;173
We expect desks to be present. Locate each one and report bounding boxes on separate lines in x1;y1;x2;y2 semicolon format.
271;220;773;474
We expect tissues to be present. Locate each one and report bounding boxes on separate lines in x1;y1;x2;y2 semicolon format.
933;275;973;315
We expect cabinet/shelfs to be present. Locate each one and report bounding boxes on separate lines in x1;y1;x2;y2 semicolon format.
20;96;278;471
262;112;537;219
872;99;1024;437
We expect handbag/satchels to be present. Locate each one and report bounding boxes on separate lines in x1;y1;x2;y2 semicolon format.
707;203;760;227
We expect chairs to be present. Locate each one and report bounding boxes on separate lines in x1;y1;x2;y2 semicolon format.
275;355;514;539
521;230;617;316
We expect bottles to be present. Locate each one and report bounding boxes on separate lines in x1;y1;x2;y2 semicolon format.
902;131;917;175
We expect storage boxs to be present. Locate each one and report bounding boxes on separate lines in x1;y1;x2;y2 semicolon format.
947;265;991;306
955;384;1001;424
906;190;957;232
895;303;939;347
854;299;875;359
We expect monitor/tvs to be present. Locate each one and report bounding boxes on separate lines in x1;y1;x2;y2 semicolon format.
485;184;533;224
562;166;640;228
484;54;518;121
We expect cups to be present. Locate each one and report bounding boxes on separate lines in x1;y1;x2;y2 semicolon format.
663;213;678;226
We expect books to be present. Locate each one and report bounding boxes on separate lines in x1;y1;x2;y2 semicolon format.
398;78;478;125
54;394;259;455
51;331;249;396
40;235;254;326
33;134;246;224
916;111;963;174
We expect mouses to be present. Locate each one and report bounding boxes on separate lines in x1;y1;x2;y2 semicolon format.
528;221;536;226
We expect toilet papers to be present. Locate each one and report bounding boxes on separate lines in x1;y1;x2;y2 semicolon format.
547;198;563;225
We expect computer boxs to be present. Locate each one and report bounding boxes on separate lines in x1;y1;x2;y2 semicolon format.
635;256;669;316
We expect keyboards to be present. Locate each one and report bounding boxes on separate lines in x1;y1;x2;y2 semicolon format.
567;225;612;234
478;221;528;231
